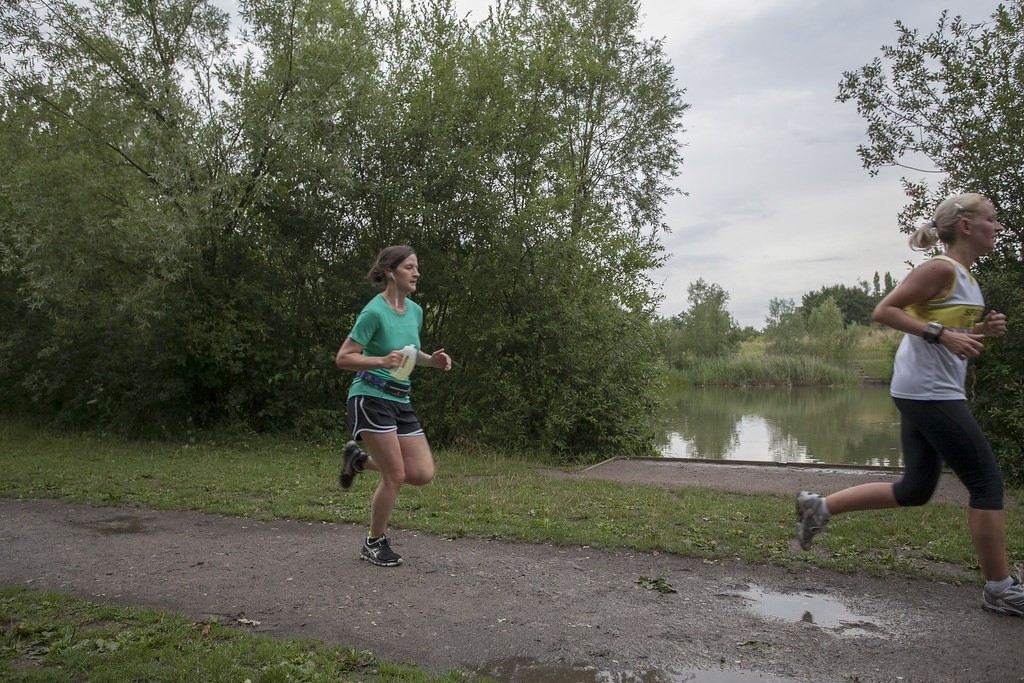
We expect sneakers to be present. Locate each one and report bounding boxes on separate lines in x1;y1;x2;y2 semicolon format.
980;571;1024;618
359;533;403;567
340;439;365;489
795;490;830;552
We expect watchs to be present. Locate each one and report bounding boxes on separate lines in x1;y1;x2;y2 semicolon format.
924;322;944;344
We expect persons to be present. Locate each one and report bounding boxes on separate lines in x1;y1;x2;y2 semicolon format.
335;246;451;567
796;193;1024;618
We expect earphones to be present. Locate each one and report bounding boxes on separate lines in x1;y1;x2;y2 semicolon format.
389;272;396;280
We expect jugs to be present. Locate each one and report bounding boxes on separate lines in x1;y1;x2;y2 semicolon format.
390;344;417;381
